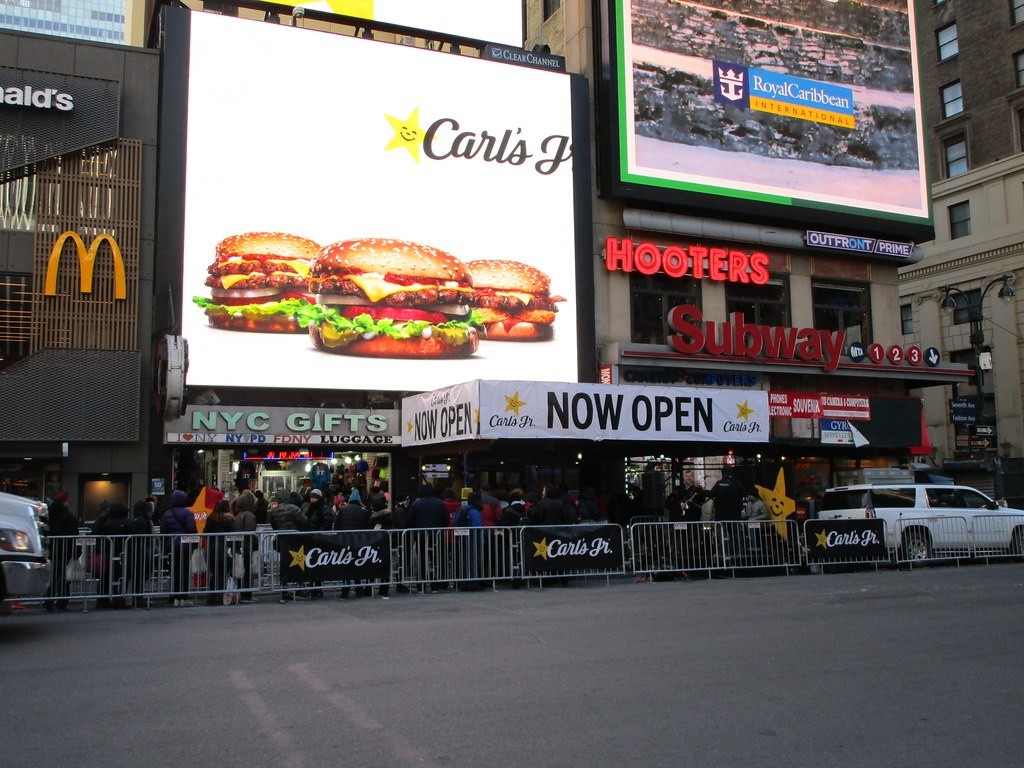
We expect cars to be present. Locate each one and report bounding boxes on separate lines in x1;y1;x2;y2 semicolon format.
0;490;51;608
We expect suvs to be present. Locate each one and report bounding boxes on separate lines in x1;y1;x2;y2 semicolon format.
817;481;1024;571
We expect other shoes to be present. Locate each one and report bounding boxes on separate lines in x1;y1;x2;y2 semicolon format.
94;577;572;612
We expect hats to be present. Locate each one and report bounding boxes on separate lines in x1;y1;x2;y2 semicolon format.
747;489;759;498
394;493;410;502
51;491;70;502
350;490;362;502
310;488;323;500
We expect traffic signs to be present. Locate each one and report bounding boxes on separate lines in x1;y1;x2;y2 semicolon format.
966;425;995;437
967;437;991;448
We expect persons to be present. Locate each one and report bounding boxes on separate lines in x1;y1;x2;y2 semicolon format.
43;461;769;611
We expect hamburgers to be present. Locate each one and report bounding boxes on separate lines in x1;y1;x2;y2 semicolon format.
191;233;567;356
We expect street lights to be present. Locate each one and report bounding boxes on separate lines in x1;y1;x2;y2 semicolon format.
939;273;1017;461
